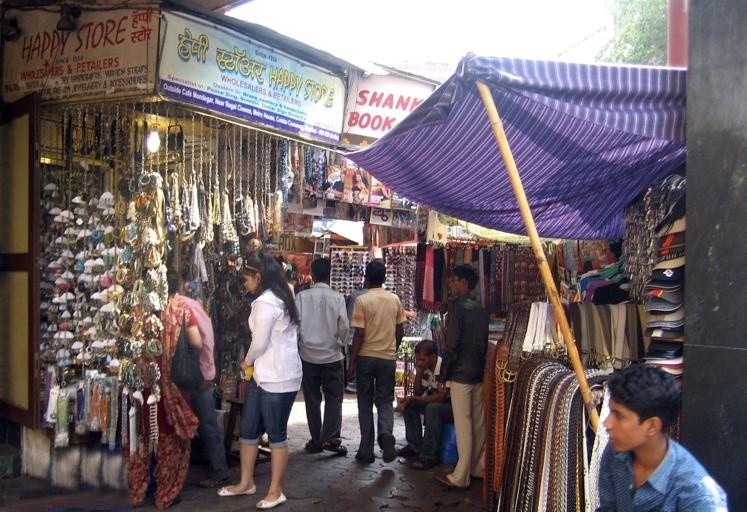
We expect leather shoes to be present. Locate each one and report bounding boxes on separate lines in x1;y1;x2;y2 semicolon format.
434;475;469;490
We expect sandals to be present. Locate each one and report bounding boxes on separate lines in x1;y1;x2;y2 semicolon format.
217;484;256;497
256;492;287;508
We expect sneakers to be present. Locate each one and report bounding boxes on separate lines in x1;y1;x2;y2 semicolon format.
382;432;397;463
197;476;231;488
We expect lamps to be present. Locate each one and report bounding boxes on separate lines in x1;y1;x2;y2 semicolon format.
146;122;161;153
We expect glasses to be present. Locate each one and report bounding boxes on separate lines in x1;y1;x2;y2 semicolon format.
331;251;364;294
385;251;417;310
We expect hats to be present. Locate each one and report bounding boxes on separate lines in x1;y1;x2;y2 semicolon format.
642;195;686;377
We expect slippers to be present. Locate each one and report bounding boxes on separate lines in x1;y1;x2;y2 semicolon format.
407;460;437;470
306;440;322;451
323;441;347;455
397;446;419;457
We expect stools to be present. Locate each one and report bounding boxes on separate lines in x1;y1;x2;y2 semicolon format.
438;422;460;467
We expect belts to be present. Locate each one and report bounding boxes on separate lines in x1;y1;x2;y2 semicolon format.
483;298;638;512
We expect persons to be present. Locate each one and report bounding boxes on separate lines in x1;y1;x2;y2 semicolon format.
370;178;391;207
350;169;370;206
216;256;302;509
594;362;731;512
331;171;347;201
392;340;455;470
123;274;202;510
169;272;232;486
293;257;350;454
434;264;488;489
346;259;407;463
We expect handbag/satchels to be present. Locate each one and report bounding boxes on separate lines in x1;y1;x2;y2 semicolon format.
169;348;203;389
